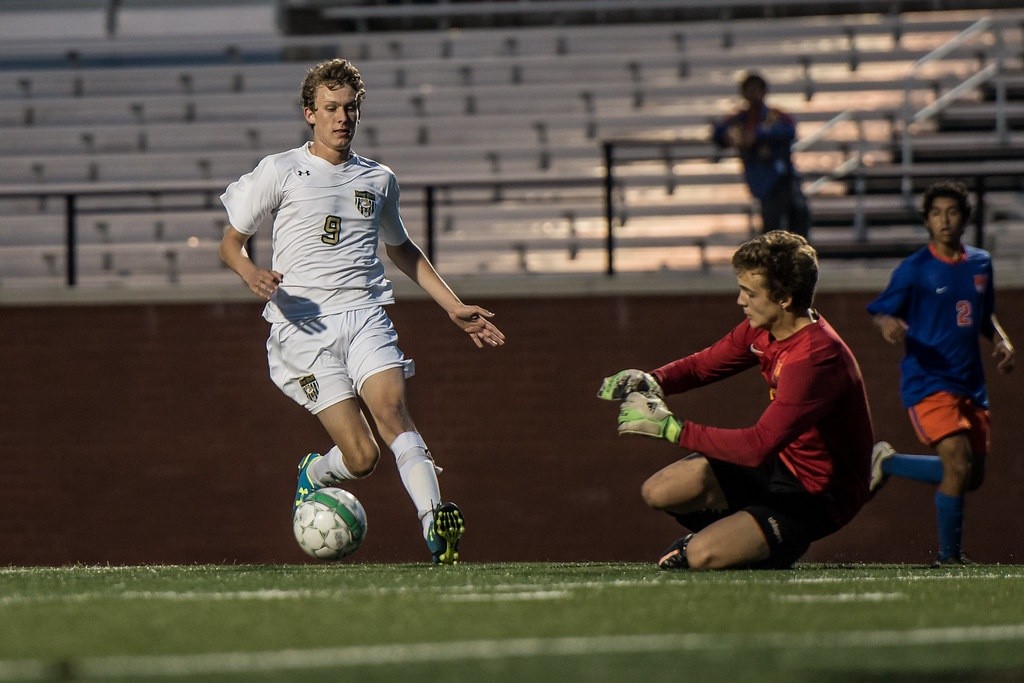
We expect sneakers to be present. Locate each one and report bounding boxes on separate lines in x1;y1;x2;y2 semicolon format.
423;499;467;566
291;452;323;520
932;550;977;567
656;532;697;569
867;441;896;494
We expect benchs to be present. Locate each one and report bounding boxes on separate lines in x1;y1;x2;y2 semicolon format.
1;9;1024;277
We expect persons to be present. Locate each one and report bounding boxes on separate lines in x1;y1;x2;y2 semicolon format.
713;76;811;238
597;230;875;571
219;59;505;565
870;179;1013;565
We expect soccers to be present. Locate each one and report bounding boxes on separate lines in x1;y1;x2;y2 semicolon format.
293;486;368;561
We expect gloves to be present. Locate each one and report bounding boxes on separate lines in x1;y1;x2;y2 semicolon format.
616;390;684;442
596;369;666;402
